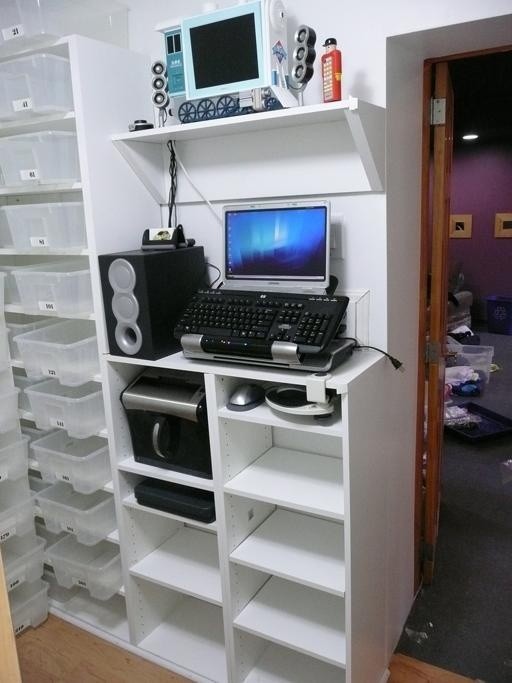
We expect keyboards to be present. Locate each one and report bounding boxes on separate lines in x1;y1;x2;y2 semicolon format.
174;288;350;354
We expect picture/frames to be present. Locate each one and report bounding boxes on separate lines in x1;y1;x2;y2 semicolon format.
494;213;512;238
449;214;472;239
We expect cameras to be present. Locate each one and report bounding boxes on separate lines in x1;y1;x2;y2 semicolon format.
129;119;154;130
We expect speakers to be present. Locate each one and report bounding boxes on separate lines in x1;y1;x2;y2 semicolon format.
151;60;170;108
99;245;209;361
292;24;316;83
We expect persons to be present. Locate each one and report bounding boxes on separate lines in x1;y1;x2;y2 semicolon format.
319;36;341;104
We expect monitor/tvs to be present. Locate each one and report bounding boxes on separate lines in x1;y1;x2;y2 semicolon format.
220;200;331;295
181;0;287;101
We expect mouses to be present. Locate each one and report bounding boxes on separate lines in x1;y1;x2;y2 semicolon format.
229;383;264;405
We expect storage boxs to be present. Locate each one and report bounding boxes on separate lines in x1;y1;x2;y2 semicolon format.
2;129;83;184
29;428;111;496
452;370;483;397
0;318;52;637
36;482;116;546
4;54;74;120
11;259;95;319
13;319;100;386
445;343;494;385
4;200;86;255
45;531;124;605
24;377;104;439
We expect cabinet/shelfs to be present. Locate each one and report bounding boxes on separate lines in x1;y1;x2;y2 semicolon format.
4;35;387;683
